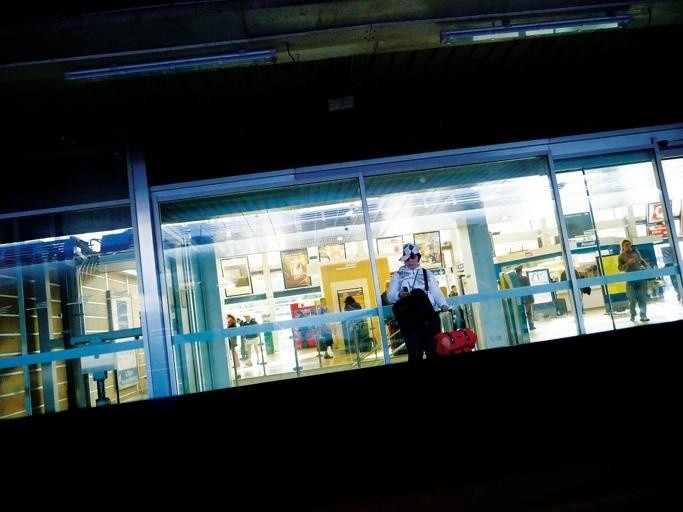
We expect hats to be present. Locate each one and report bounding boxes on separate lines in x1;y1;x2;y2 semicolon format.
344;296;355;303
514;265;523;271
399;243;419;261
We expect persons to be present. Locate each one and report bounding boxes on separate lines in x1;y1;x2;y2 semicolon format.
296;311;311;349
317;297;334;358
387;242;450;362
560;268;586;314
343;262;537;356
617;239;653;321
228;314;274;368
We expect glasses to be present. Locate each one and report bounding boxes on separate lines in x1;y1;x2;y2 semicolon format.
407;254;419;261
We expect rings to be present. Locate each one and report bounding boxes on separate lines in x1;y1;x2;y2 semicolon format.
404;294;406;296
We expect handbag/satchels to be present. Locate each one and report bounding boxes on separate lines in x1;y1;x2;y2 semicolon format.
434;328;477;356
628;266;642;289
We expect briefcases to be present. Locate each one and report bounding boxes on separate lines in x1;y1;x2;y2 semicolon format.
319;337;327;351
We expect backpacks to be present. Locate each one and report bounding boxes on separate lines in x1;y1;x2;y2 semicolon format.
393;288;440;351
